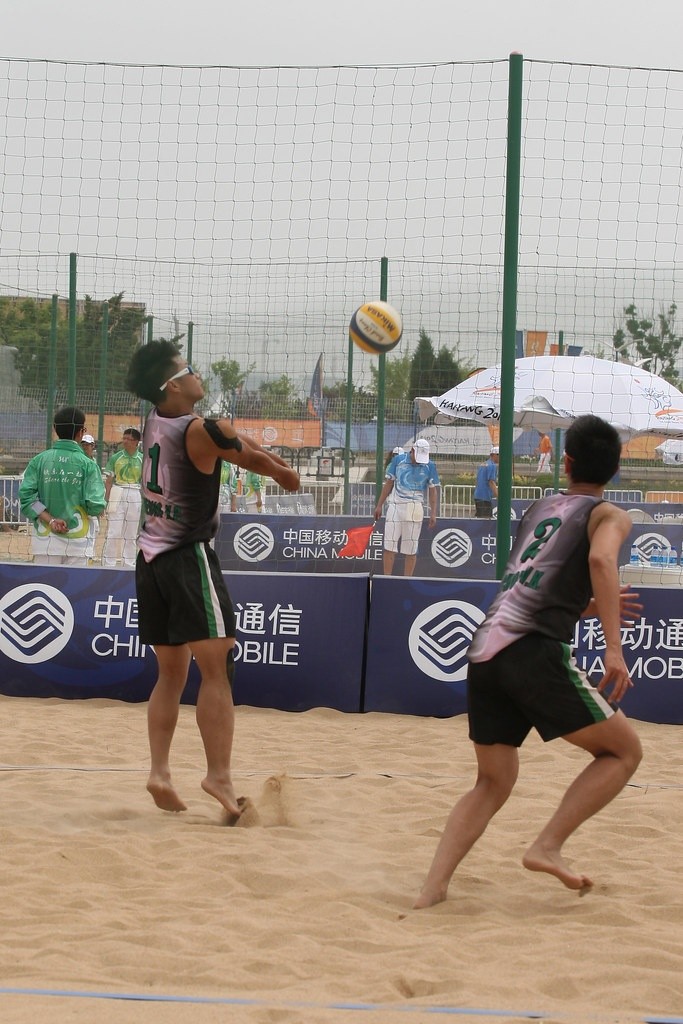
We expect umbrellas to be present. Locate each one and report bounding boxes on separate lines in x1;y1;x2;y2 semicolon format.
413;355;683;498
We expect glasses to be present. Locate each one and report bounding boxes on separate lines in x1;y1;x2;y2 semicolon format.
158;364;194;392
122;437;135;441
82;427;87;433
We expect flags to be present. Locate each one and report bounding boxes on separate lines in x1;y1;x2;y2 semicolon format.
337;526;374;559
307;353;322;417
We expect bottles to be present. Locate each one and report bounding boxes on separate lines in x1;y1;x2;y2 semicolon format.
650;545;677;568
630;545;639;566
680;550;683;567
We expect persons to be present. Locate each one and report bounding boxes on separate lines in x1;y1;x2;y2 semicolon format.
472;446;499;519
373;438;441;576
534;430;553;476
218;459;265;515
118;337;301;818
18;407;144;569
404;412;643;909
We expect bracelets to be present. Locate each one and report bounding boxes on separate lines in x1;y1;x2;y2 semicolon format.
49;518;57;525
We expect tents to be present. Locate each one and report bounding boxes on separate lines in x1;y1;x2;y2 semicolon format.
654;438;683;467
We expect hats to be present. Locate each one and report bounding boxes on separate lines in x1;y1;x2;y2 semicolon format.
393;447;404;455
413;439;429;463
81;435;94;443
490;446;499;454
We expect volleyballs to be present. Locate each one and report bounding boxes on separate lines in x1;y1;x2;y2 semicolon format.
348;300;404;356
